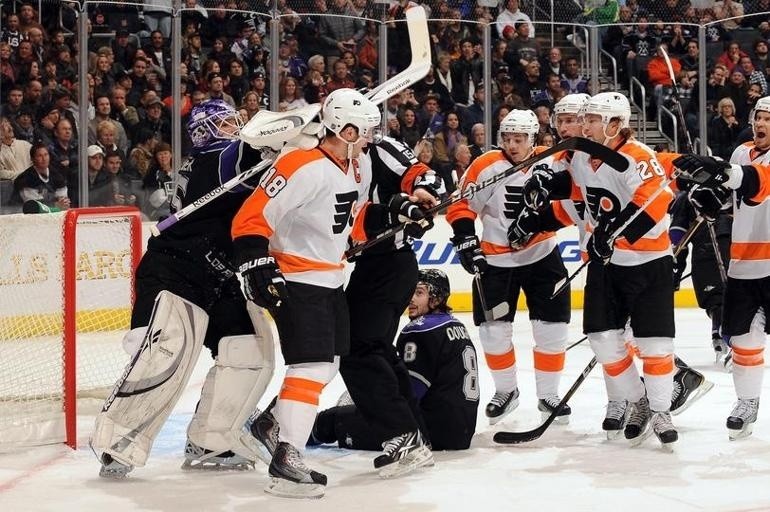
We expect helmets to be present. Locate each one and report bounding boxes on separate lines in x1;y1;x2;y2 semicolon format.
321;88;381;144
187;100;245;148
500;109;539;146
577;92;631;139
414;269;450;308
554;93;591;126
752;97;770;139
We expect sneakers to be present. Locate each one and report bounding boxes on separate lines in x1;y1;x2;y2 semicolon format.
602;358;705;442
727;396;759;428
374;430;432;469
101;452;126;470
486;387;519;416
269;442;328;485
250;410;280;455
711;311;734;369
539;396;571;415
185;440;249;465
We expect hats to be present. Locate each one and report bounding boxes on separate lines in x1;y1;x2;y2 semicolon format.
279;33;296;46
88;145;105;157
144;96;165;107
208;72;223;82
106;143;120;156
477;63;513;89
115;69;133;80
116;28;129;36
248;72;263;81
503;18;527;33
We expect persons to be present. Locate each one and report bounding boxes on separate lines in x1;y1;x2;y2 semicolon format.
0;1;769;217
91;88;770;497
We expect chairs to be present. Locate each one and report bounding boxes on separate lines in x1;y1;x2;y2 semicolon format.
636;30;761;79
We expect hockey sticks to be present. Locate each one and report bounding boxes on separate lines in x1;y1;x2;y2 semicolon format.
341;137;629;260
551;169;683;296
493;217;703;443
151;5;431;236
474;265;511;323
660;46;726;284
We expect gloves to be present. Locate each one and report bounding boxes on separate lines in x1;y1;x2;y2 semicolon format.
239;256;289;309
449;233;488;273
522;163;557;211
507;207;545;250
587;217;615;264
673;267;680;291
388;193;434;239
670;154;732;218
